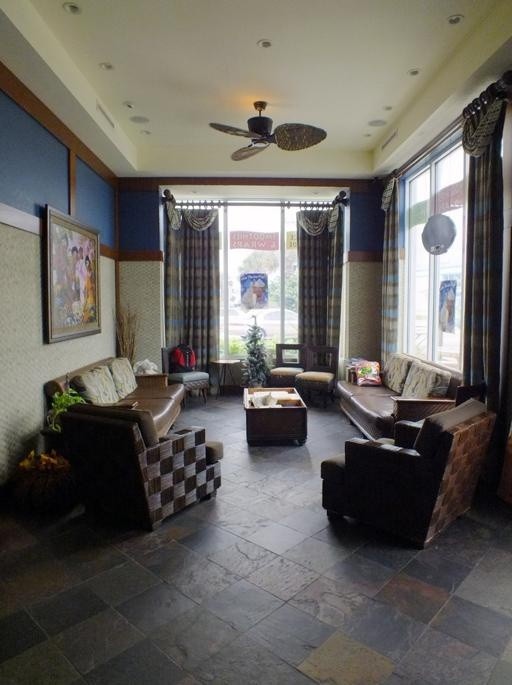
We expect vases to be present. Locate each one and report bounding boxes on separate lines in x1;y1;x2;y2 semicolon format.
26;493;53;518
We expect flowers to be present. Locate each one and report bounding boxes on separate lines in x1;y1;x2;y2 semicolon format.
14;449;75;495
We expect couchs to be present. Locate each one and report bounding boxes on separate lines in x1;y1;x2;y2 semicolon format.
40;357;186;452
334;352;463;442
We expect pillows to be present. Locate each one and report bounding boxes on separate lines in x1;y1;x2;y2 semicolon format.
381;353;420;393
66;405;158;448
355;361;382;386
73;364;118;407
107;357;139;398
414;398;484;450
402;360;451;399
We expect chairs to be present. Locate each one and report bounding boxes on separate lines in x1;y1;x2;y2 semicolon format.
167;344;338;409
60;404;223;531
320;397;497;550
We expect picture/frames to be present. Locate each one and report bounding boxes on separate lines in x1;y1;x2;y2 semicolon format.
44;204;101;344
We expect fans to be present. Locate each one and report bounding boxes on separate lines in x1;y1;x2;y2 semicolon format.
210;101;326;161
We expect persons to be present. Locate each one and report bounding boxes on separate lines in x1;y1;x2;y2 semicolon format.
55;234;94;319
